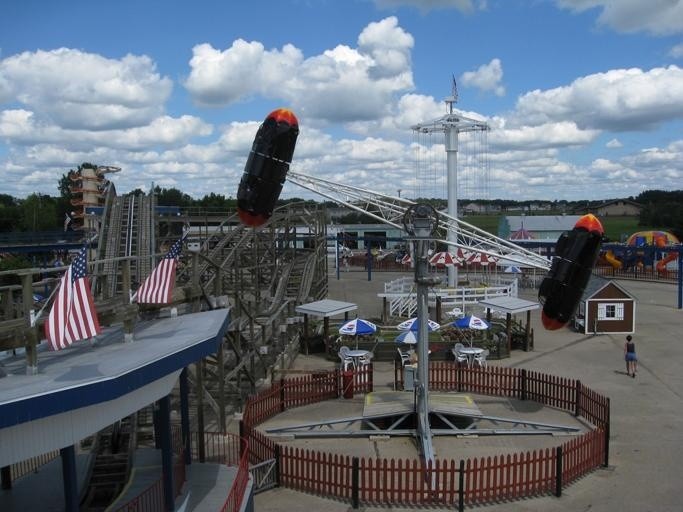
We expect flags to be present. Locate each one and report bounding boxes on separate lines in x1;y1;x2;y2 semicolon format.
135;239;182;303
44;246;101;351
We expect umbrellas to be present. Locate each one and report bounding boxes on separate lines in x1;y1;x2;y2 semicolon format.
503;266;521;279
394;330;418;352
337;316;376;350
396;316;440;334
395;248;498;287
449;314;491;347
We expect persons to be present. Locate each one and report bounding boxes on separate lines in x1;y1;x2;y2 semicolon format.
624;334;637;377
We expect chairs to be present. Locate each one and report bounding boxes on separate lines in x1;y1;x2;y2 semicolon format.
397;347;432;379
452;343;488;371
338;346;374;372
